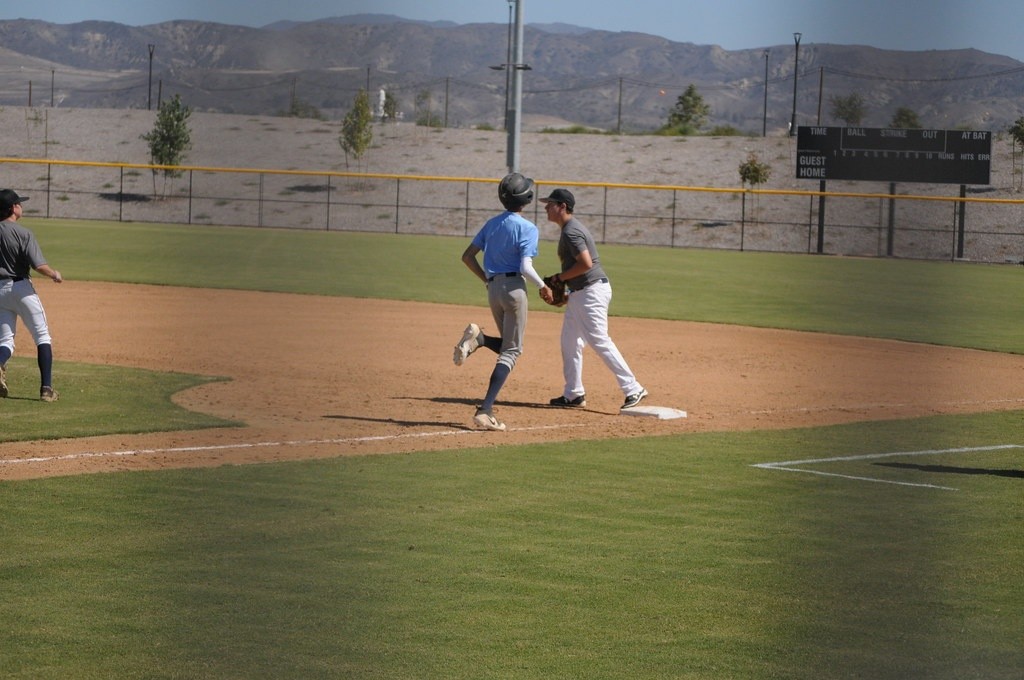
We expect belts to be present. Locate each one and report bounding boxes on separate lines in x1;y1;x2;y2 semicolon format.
13;277;24;282
489;272;516;281
571;278;607;292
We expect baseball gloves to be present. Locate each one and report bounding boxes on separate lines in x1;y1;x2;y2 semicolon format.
540;274;570;308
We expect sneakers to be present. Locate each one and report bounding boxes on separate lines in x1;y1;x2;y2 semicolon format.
454;323;484;366
550;395;586;407
621;389;647;408
40;385;59;402
0;368;9;397
473;406;507;431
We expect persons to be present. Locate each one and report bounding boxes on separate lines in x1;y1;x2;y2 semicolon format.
540;188;648;409
452;173;553;431
0;188;62;402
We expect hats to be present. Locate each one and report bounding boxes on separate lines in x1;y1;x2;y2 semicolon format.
538;190;576;211
0;189;30;207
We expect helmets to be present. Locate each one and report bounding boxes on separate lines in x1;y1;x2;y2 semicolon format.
499;172;533;204
